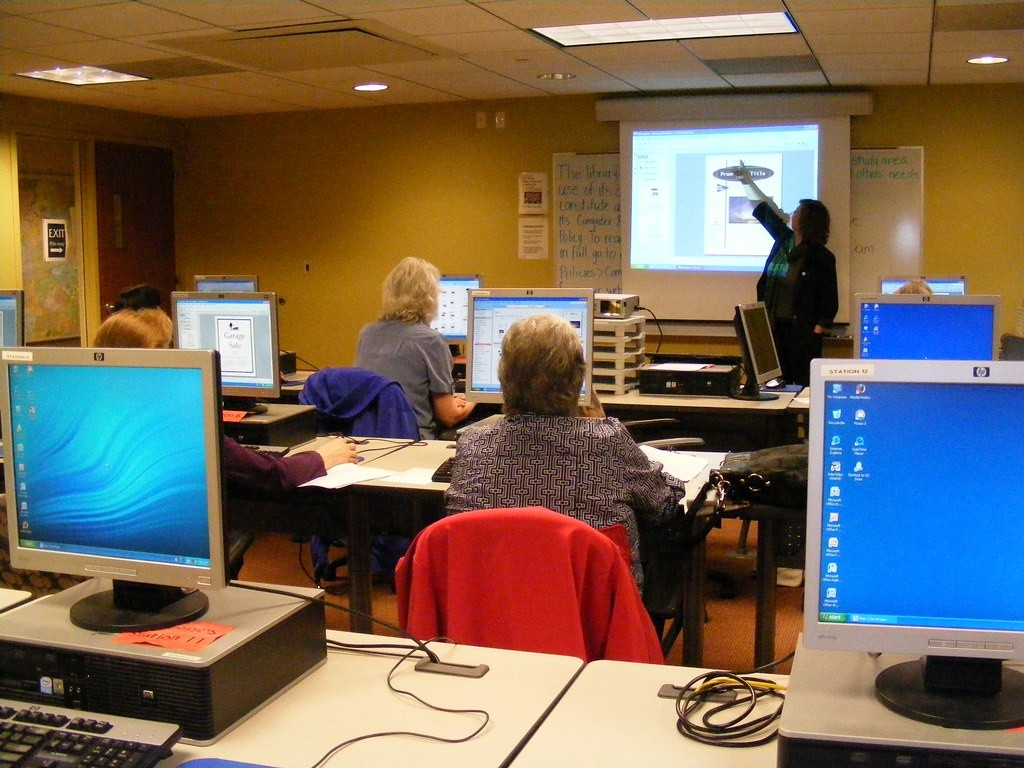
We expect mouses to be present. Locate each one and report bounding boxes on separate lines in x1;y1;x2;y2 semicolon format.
765;378;785;388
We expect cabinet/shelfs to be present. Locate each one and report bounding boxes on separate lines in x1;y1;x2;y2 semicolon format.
594;315;647;395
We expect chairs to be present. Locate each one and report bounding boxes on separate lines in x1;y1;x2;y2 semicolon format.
384;504;686;664
574;385;747;597
298;362;429;585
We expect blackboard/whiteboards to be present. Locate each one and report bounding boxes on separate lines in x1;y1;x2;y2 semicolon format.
552;145;925;324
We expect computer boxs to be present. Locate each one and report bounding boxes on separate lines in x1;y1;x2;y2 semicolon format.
774;634;1024;768
279;351;296;377
633;363;740;398
0;579;328;747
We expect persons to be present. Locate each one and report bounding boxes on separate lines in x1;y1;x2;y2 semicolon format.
733;159;839;440
112;282;162;309
93;308;358;524
893;278;932;294
443;310;685;649
353;256;477;441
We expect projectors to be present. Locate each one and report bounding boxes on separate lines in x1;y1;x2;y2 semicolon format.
594;292;640;320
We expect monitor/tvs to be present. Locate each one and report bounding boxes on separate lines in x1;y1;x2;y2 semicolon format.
802;276;1024;729
428;271;484;357
465;288;594;407
732;305;783;400
0;275;282;633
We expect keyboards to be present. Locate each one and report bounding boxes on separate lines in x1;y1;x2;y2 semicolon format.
0;698;184;768
454;378;466;393
431;456;455;483
240;444;289;458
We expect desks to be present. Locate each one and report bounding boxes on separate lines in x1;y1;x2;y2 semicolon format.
0;369;811;768
